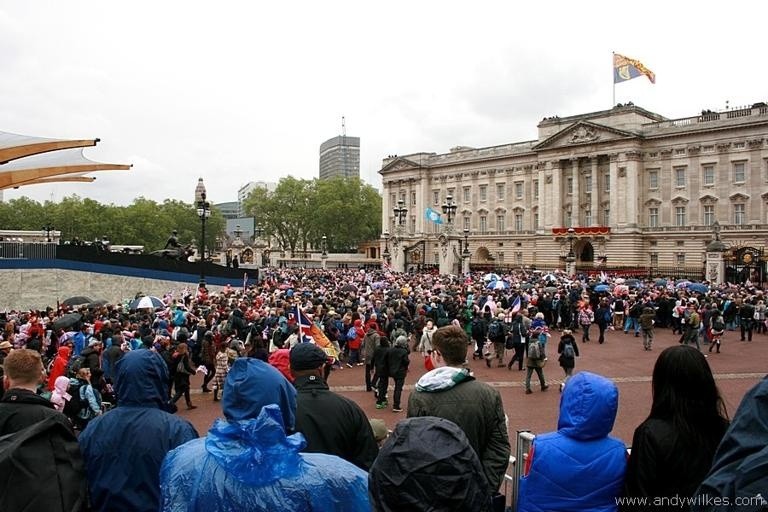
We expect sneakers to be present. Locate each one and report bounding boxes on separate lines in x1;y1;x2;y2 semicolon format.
558;383;565;393
346;363;353;368
508;363;511;370
392;406;404;412
519;368;525;370
357;362;364;366
472;350;491;360
370;383;388;409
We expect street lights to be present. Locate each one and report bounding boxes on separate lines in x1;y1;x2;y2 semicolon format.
711;221;721;240
394;199;407;225
565;228;576;255
194;191;211;279
382;228;390;253
440;194;455;223
461;227;470;254
42;221;54;243
320;234;327;255
235;224;240;237
257;222;262;236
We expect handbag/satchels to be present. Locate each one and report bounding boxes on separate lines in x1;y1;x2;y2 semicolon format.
198;352;204;365
604;313;612;324
521;336;526;343
177;355;192;375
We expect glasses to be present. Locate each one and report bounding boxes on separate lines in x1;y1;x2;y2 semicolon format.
426;349;442;355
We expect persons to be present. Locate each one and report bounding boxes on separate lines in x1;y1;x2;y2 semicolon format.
0;229;768;512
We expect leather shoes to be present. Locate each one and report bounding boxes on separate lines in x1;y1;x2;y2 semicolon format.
541;383;548;391
486;358;491;368
201;383;212;392
366;388;372;392
525;389;533;394
498;362;506;367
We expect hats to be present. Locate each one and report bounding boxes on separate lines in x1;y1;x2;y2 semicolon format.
369;418;390;440
289;343;328;369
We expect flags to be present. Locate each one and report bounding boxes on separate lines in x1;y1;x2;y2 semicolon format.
614;53;655;85
425;208;443;225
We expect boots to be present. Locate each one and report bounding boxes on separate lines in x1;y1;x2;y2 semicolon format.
213;386;221;401
186;401;197;409
709;339;716;352
716;343;721;353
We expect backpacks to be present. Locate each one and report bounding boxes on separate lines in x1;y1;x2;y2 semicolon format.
64;385;88;420
488;321;503;340
347;327;359;340
274;328;284;347
565;343;575;359
506;336;514;350
528;340;542;359
41;391;52;401
471;318;481;341
